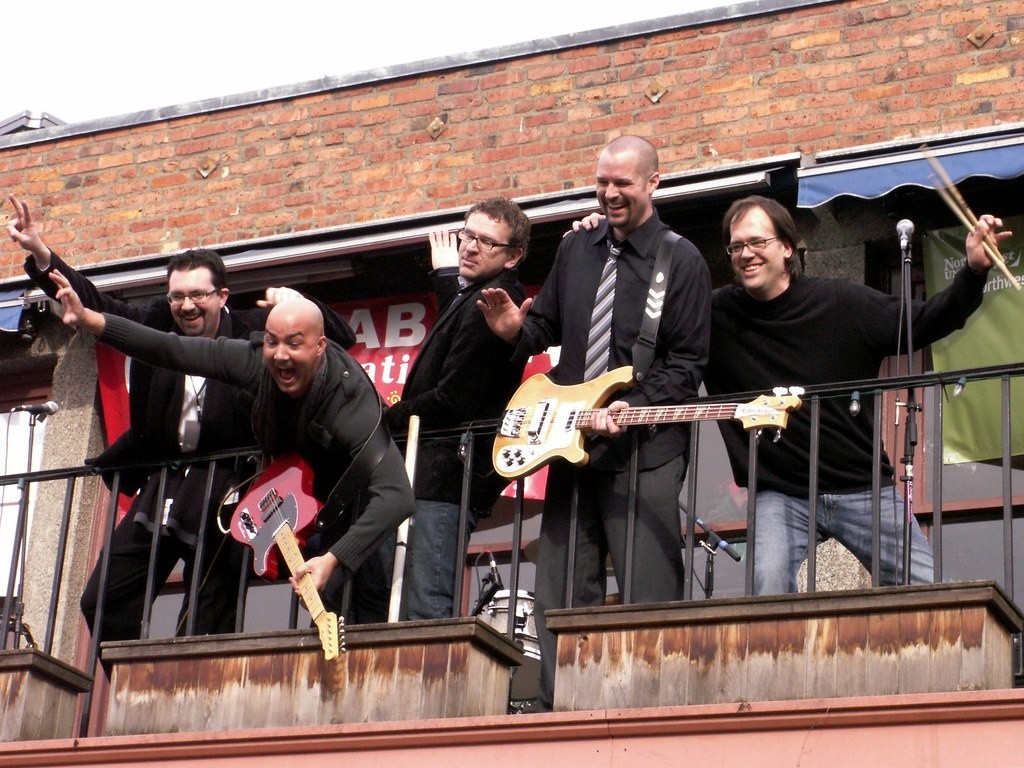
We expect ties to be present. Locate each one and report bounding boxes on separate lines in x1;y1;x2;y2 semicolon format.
584;239;623;441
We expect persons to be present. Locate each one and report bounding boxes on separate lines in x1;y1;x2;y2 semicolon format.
8;193;357;686
347;195;529;623
476;136;712;714
47;267;417;632
563;196;1014;601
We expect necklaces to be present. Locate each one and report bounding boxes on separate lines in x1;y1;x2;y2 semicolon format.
190;375;207;423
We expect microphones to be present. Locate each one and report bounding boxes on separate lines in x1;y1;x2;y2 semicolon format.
896;218;915;250
10;401;59;415
488;552;503;590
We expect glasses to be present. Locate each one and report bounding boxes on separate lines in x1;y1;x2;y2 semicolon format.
456;228;514;252
724;236;780;255
166;289;219;305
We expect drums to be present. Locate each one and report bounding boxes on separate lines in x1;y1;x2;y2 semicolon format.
476;588;543;663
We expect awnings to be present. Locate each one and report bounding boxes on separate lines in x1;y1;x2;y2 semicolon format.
796;131;1024;210
0;287;30;333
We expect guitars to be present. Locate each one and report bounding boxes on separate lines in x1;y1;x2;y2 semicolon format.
491;365;805;482
229;452;346;665
